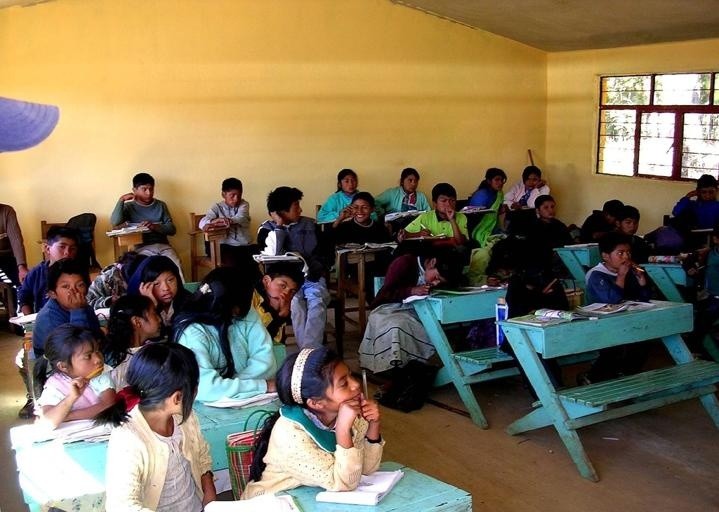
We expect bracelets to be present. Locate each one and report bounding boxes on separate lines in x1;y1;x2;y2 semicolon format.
364;433;381;443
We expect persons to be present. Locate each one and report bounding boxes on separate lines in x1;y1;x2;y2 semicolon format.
107;171;185;283
255;185;336;352
198;176;252;268
89;337;217;512
0;203;30;338
238;345;386;503
468;165;574;311
316;168;469;305
579;174;717;361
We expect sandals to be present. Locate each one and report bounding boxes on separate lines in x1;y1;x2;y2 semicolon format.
372;383;387;400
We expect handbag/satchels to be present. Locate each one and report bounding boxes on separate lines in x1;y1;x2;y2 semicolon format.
499;272;570;357
226;409;278;501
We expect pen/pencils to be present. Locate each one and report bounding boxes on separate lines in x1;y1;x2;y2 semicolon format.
697;265;705;270
629;264;645;271
85;366;104;379
542;277;558;293
362;370;368;399
601;436;620;442
419;224;435;237
216;203;227;219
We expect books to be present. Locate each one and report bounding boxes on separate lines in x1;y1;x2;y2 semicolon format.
384;205;495;223
105;220;151;238
315;470;404;506
250;250;309;278
402;280;507;305
507;297;657;329
204;391;280;409
203;492;305;512
335;232;447;255
13;418;115;445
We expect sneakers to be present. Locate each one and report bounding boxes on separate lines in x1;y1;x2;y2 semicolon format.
19;398;34;418
